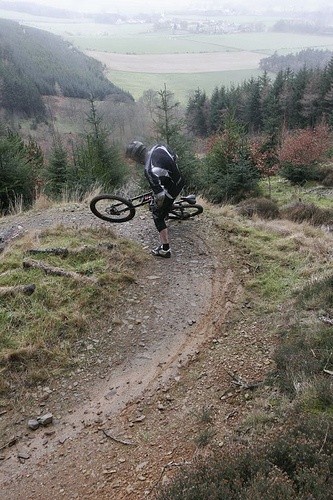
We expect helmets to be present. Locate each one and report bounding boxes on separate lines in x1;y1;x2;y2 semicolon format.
125;141;146;160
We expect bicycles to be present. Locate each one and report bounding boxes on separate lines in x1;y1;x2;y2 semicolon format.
89;186;203;223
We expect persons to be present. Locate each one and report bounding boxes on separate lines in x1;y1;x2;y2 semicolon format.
125;140;185;259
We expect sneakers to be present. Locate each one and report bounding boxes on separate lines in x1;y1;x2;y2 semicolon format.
151;244;172;259
